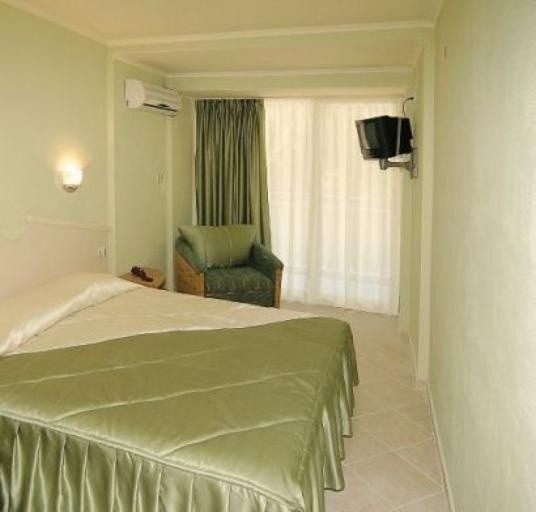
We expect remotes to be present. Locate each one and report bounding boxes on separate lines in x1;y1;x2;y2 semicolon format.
145;271;153;282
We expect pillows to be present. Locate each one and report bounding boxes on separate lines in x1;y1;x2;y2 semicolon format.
177;222;257;268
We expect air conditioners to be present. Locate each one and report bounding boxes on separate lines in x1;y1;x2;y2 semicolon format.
123;78;183;120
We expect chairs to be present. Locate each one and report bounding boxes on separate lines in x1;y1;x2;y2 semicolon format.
173;236;283;310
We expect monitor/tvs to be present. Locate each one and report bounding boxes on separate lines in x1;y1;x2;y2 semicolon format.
355;115;413;160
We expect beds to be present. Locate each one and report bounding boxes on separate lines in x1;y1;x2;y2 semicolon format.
0;215;358;512
